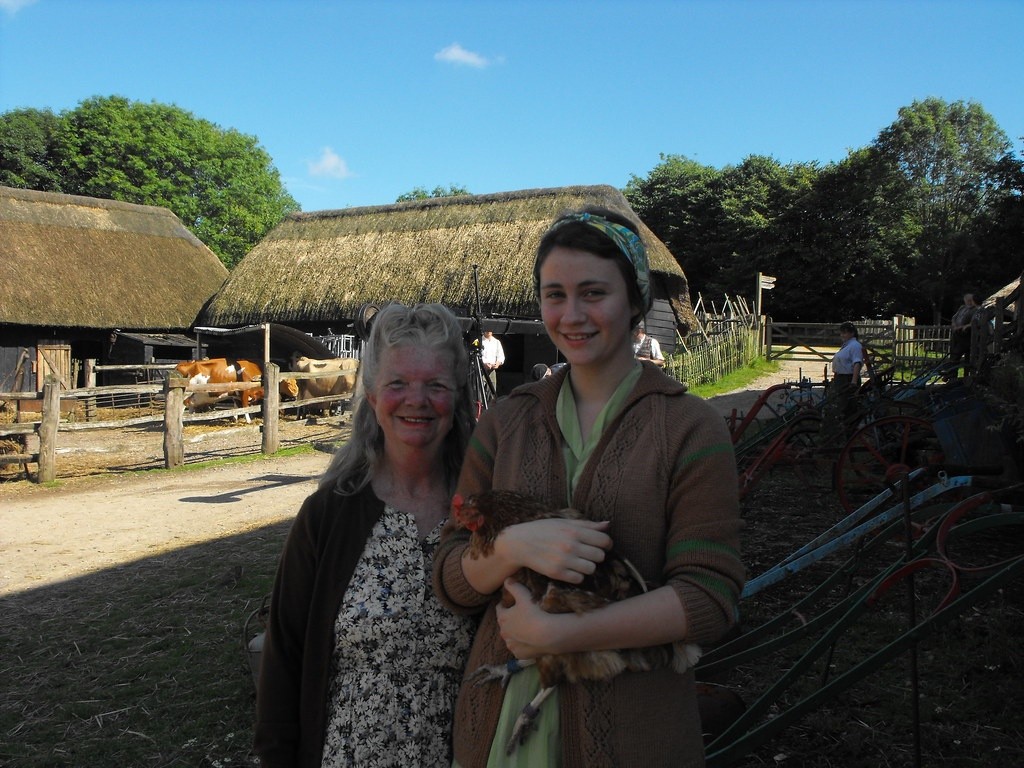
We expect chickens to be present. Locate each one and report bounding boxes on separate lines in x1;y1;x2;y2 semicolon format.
451;488;704;759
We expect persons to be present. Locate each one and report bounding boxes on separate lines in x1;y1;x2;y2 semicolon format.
434;205;744;768
949;293;977;378
531;362;567;381
250;303;477;768
473;331;506;392
632;321;666;366
832;322;864;422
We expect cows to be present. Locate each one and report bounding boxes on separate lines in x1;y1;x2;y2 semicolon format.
174;358;299;425
287;350;361;420
